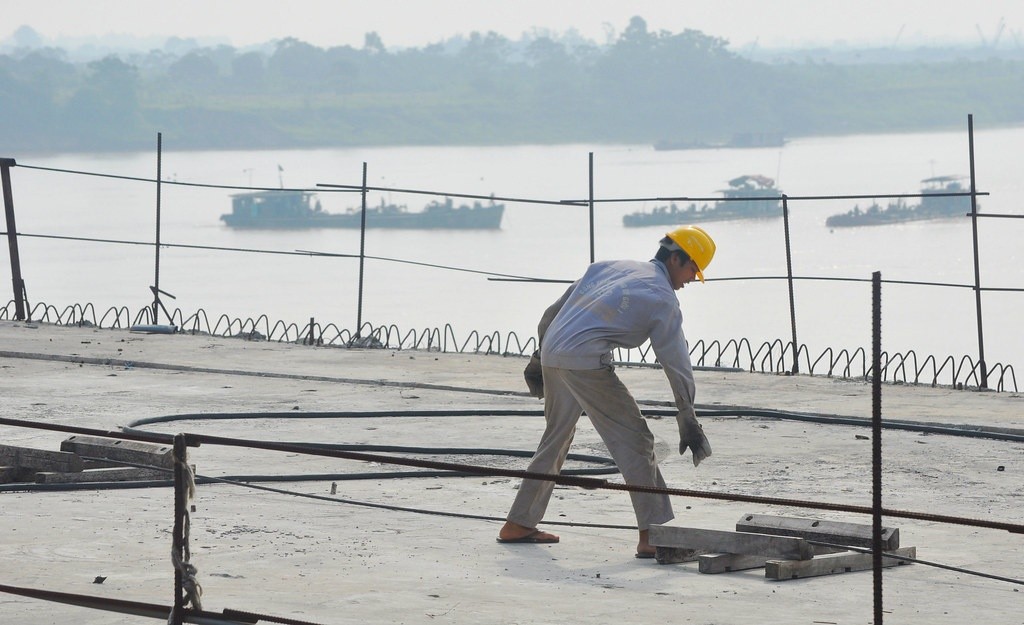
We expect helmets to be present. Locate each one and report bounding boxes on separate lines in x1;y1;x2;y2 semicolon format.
665;224;716;283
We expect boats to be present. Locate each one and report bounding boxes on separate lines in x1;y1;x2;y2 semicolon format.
622;174;790;228
824;175;981;227
218;163;505;230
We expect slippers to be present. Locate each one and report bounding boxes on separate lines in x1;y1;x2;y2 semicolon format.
635;551;654;557
496;528;559;543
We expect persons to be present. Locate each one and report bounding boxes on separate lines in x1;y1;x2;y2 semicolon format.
496;227;716;559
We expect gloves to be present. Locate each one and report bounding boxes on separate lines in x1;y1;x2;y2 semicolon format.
675;406;711;466
524;349;543;398
